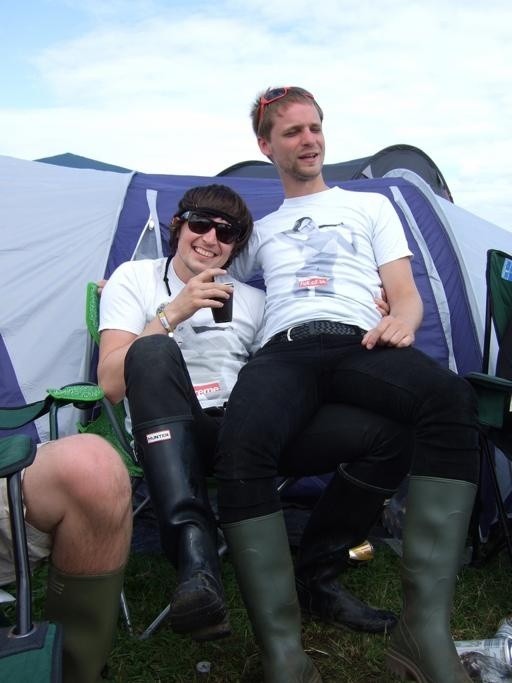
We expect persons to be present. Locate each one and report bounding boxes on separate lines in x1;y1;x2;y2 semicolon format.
95;84;484;683
96;182;397;641
0;432;135;683
274;216;358;298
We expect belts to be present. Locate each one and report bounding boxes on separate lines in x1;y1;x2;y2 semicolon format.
261;322;369;348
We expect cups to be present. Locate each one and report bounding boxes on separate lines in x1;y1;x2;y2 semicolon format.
208;282;234;324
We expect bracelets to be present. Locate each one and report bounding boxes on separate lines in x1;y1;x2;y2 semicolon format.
155;302;177;332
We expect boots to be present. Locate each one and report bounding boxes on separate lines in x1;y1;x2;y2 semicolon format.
218;505;322;683
132;414;234;641
297;460;397;635
383;476;477;683
43;563;124;683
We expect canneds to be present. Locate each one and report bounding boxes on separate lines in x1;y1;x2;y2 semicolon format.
349;540;374;563
482;614;512;682
453;637;512;669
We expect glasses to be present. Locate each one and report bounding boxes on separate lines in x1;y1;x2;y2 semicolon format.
179;211;242;245
257;87;315;134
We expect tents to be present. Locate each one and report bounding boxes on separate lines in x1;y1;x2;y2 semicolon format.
0;144;512;569
